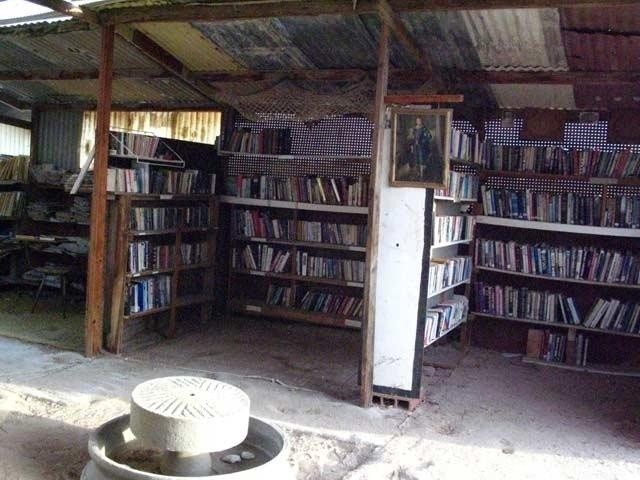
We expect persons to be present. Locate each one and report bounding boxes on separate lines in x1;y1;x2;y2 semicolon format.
409;117;432;179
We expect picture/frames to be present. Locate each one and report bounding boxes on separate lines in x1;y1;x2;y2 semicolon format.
390;108;452;188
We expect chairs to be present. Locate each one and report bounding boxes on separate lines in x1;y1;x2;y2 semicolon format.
16;235;82;318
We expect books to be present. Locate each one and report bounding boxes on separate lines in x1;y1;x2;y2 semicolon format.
221;129;367;321
106;131;216;313
0;155;94;293
422;128;640;366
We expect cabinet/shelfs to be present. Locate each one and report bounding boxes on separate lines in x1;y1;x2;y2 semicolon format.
0;183;93;296
218;137;369;334
419;134;477;353
468;145;640;353
106;132;218;354
